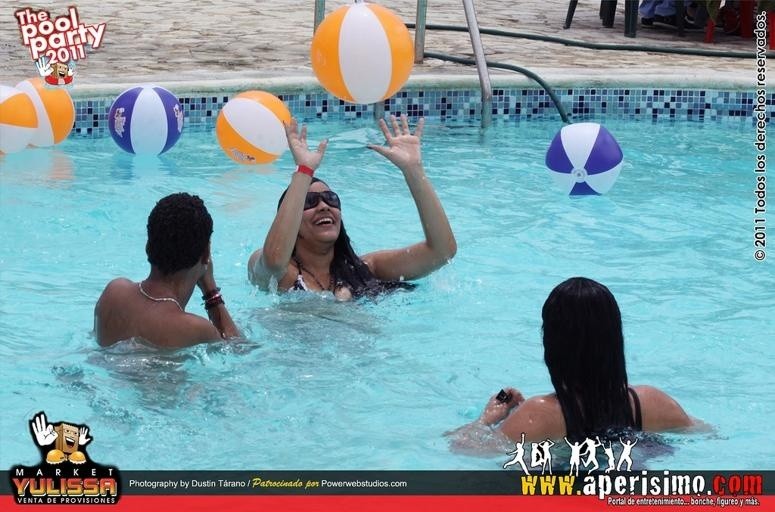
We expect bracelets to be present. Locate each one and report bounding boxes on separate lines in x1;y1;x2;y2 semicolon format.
203;286;225;308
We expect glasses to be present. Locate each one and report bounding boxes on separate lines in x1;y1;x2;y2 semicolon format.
304;192;340;210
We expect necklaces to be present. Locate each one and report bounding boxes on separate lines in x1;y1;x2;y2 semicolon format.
296;261;337;293
138;279;185;312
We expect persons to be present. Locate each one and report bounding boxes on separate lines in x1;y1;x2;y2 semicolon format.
639;0;725;34
248;113;458;301
93;192;247;353
445;275;719;459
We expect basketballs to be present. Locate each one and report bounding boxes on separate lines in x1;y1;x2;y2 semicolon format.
218;85;296;165
0;81;40;161
309;3;416;106
107;84;187;155
19;73;78;151
